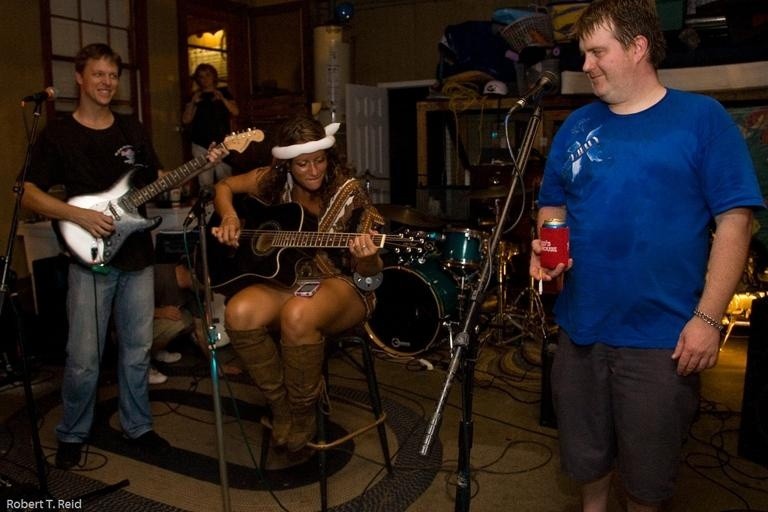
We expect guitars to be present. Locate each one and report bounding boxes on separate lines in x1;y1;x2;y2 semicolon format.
51;127;264;271
195;193;431;296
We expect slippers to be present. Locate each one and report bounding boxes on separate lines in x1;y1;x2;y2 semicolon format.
180;363;224;379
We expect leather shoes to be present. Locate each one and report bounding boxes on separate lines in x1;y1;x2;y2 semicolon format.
137;431;168;453
55;442;84;469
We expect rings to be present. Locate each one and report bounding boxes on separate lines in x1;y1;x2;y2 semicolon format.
360;245;366;249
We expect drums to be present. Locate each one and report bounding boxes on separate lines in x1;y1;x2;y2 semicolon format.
363;262;463;358
439;227;492;270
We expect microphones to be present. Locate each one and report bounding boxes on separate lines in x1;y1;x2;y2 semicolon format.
183;184;216;226
23;86;58;104
515;70;560;108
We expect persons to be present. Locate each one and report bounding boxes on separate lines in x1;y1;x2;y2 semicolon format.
210;114;388;454
528;1;767;512
180;63;240;199
12;42;230;470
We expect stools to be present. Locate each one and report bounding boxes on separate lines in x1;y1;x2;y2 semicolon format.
260;327;393;511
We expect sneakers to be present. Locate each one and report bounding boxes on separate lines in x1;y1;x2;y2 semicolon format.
148;368;167;384
155;350;181;363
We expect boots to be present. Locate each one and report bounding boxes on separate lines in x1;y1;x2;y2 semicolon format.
224;328;292;447
279;335;325;453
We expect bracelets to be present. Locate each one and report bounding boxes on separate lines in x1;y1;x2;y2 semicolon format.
693;306;724;332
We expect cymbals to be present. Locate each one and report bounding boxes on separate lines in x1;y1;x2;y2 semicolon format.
372;204;446;227
466;184;534;199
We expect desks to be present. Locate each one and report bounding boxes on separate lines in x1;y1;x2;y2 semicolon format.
16;198;214;315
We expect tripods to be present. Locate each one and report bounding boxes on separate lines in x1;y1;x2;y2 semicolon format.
0;272;129;512
